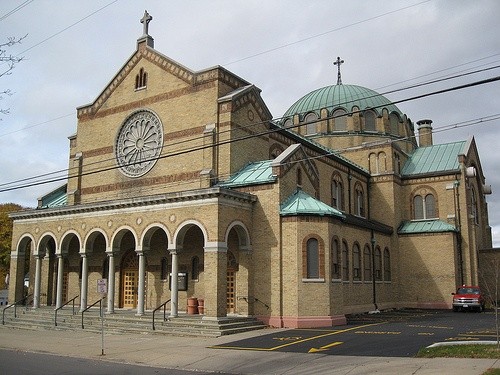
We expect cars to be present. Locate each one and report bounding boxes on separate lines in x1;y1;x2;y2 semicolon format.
451;284;487;312
